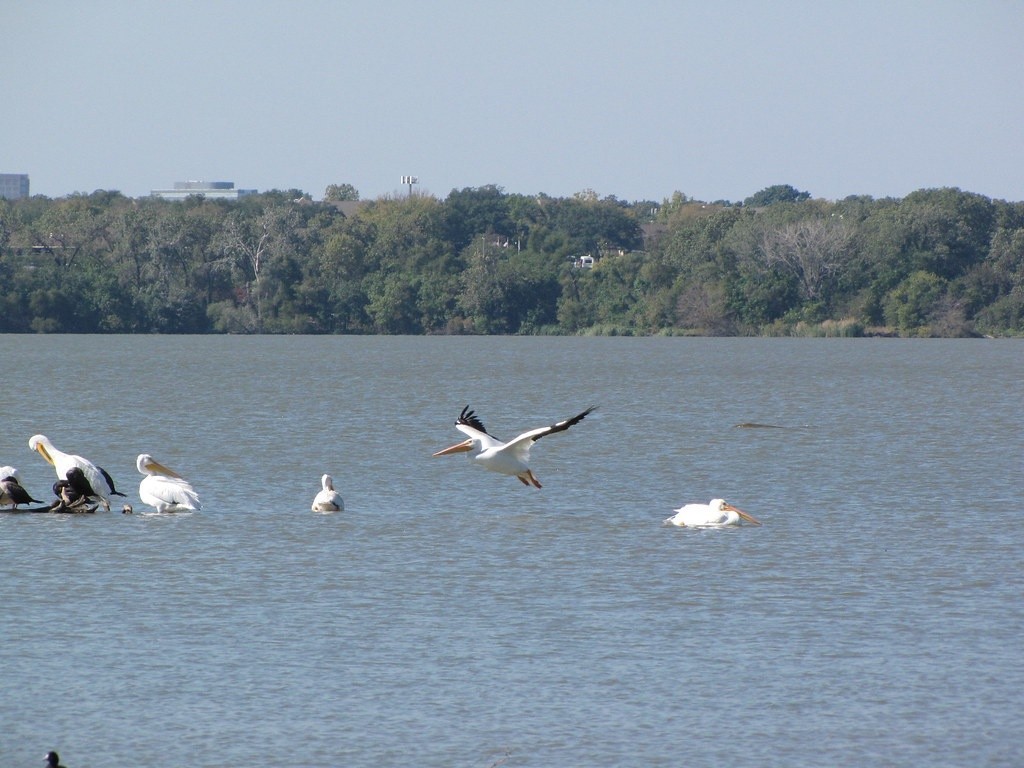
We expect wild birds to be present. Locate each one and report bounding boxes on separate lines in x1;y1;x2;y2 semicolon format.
311;473;345;512
432;403;602;490
0;434;205;514
663;498;762;527
